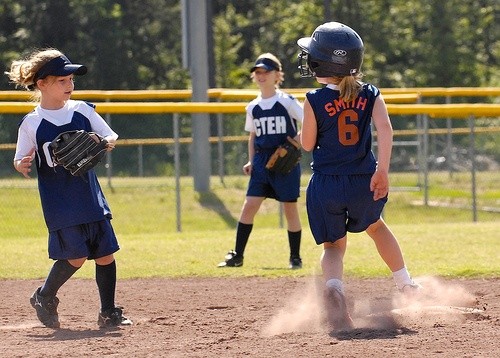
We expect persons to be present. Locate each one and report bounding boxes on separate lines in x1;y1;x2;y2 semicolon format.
217;53;302;269
296;20;423;334
5;48;134;328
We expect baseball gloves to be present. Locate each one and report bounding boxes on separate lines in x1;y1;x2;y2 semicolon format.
265;136;302;177
49;129;109;178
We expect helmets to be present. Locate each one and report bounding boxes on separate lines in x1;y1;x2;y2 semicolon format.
297;22;364;78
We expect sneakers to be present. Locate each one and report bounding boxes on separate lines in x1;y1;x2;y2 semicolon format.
399;281;424;299
323;286;355;332
98;308;132;325
30;286;60;328
218;250;243;267
289;258;302;269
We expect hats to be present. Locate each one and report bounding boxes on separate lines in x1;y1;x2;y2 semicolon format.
27;55;88;91
250;58;279;73
